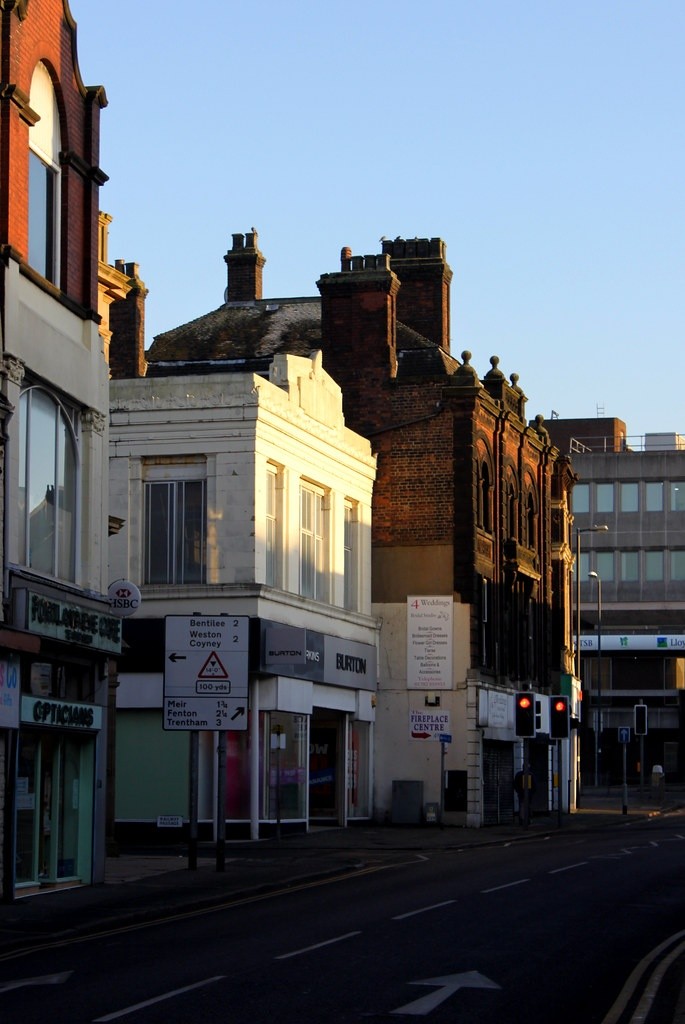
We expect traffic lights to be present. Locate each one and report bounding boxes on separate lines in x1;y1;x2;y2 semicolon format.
634;705;647;736
514;692;536;738
550;695;570;740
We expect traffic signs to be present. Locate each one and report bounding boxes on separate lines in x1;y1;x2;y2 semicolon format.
162;614;249;733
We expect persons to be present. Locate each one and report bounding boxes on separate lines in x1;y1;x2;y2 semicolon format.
514;763;534;826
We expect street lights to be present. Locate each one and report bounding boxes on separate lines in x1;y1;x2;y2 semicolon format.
588;570;602;789
577;524;609;795
